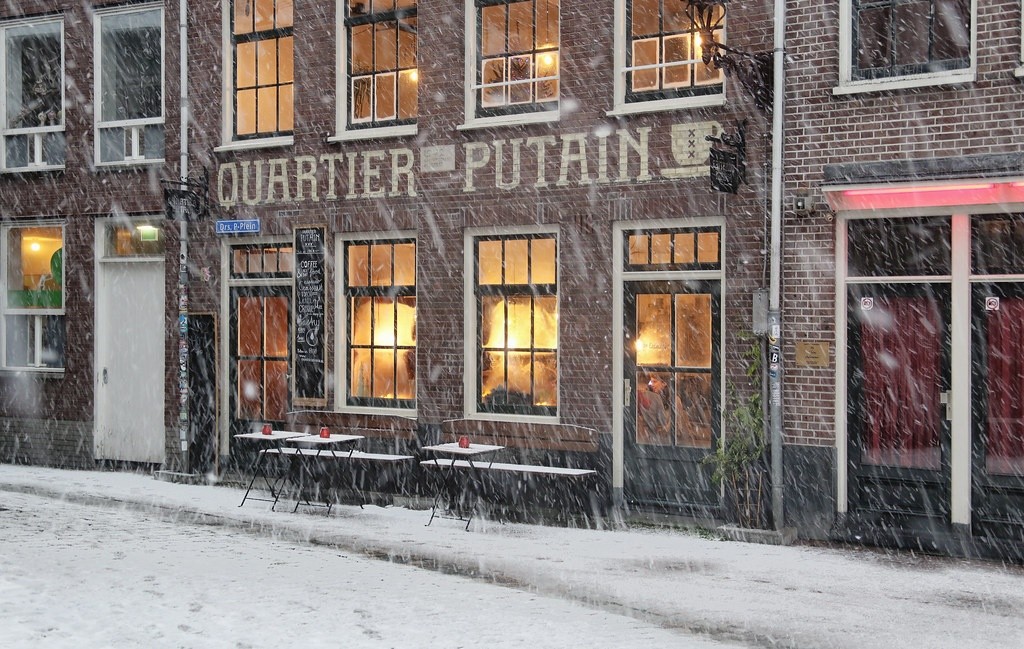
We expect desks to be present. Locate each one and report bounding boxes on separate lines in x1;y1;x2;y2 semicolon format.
285;433;365;517
234;430;310;512
421;441;506;532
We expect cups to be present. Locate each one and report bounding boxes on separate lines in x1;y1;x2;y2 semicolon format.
458;435;469;448
320;427;330;438
262;423;272;435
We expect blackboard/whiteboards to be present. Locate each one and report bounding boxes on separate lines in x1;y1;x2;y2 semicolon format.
293;224;329;408
187;313;220;475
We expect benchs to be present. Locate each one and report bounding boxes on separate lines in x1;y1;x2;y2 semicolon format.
420;418;599;526
255;410;419;506
